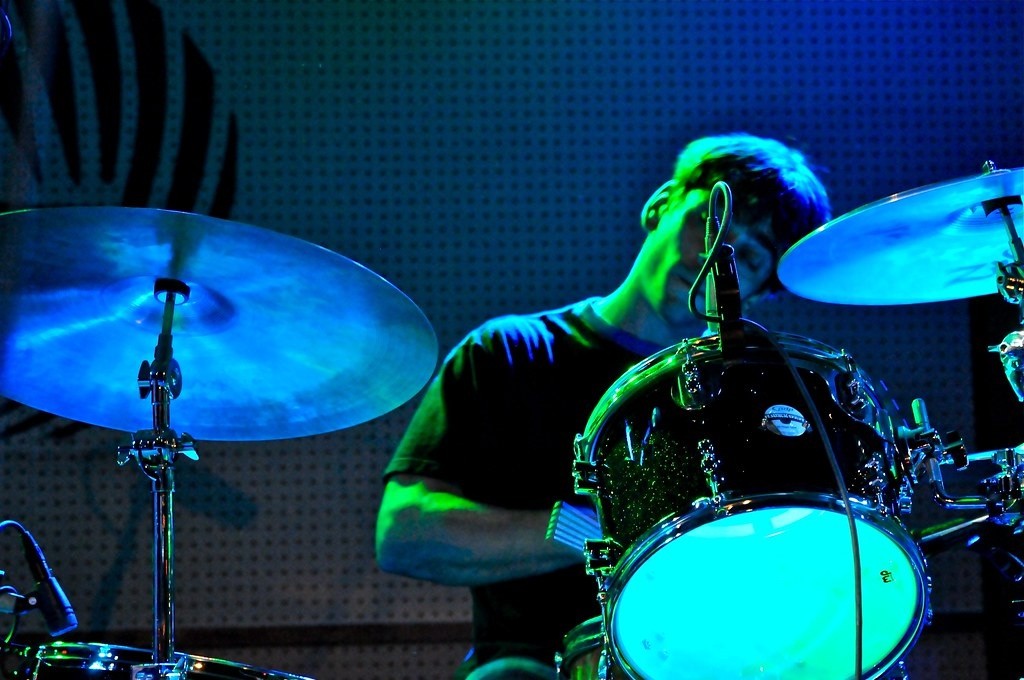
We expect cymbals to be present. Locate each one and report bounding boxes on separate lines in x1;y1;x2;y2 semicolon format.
0;204;440;442
777;169;1024;305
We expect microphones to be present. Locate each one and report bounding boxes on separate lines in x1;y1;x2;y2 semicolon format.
704;215;742;332
22;531;77;636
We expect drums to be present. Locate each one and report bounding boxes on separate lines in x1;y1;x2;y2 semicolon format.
565;614;630;680
574;332;934;680
32;640;317;680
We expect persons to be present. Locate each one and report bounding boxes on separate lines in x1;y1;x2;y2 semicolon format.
375;132;847;680
